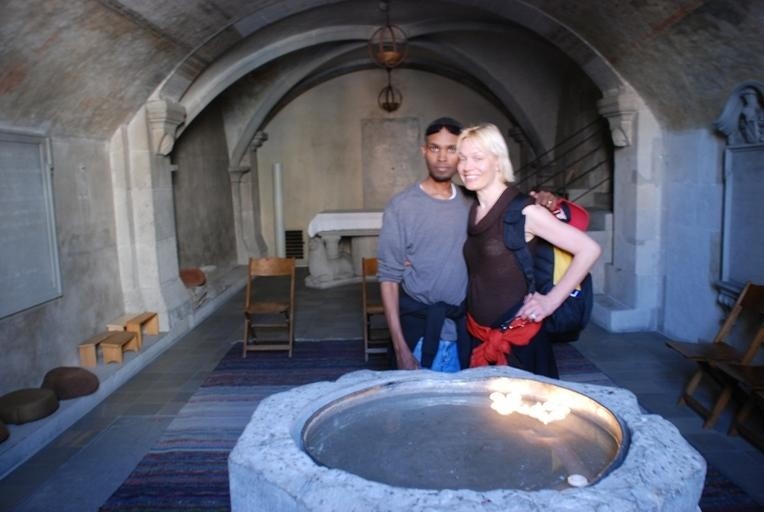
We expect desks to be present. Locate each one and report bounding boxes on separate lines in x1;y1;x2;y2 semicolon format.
303;209;383;289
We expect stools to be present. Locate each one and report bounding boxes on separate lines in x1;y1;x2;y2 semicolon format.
76;310;160;367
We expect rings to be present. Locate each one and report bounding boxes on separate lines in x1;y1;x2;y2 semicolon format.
546;199;552;204
531;314;536;319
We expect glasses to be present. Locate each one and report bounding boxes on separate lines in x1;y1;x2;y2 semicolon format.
425;124;461;136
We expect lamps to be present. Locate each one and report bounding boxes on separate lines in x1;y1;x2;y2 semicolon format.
367;0;409;112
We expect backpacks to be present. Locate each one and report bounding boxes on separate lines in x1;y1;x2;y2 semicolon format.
503;193;593;343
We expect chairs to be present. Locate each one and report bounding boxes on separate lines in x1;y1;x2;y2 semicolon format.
360;256;389;362
241;255;296;359
663;280;763;454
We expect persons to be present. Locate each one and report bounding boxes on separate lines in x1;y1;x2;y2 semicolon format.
376;117;590;369
454;122;601;379
